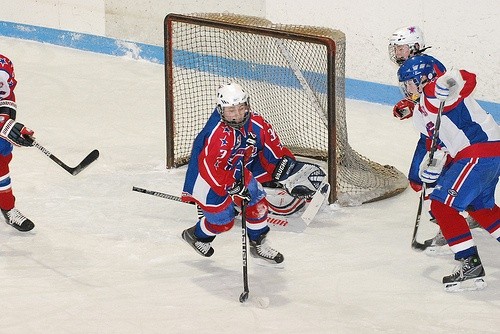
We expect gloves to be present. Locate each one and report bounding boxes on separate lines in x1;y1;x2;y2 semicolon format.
0;118;36;147
434;66;465;101
226;181;251;208
0;99;17;120
418;150;447;183
393;99;414;120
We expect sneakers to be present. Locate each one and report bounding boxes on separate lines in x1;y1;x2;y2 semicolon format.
248;227;284;269
1;207;35;233
181;223;214;258
425;229;453;255
443;254;488;291
467;215;484;231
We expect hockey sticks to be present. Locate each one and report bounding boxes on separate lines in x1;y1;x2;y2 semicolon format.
132;182;331;233
239;160;249;303
411;101;434;252
21;133;99;177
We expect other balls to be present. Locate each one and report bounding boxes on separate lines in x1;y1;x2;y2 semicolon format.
255;295;270;309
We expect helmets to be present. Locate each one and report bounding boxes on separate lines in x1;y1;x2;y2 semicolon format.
216;82;251;127
388;25;423;65
397;56;436;103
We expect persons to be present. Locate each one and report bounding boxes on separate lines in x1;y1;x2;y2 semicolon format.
180;83;326;269
0;54;36;233
387;24;500;293
